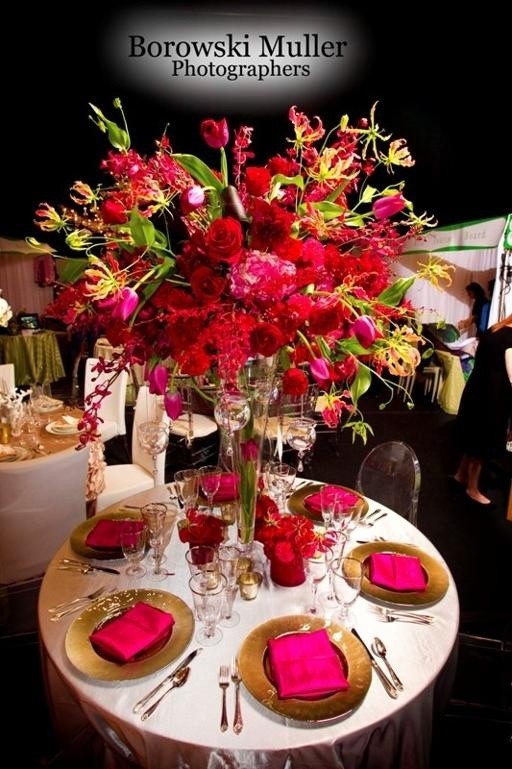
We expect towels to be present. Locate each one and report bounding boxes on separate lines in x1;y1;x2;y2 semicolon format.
52;415;79;433
194;474;242;503
305;487;358;514
268;629;349;699
370;552;426;592
85;520;145;548
90;602;174;664
0;445;16;462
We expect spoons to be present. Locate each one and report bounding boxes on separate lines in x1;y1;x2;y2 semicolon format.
141;667;191;722
371;637;404;691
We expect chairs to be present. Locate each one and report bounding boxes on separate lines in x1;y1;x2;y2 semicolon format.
357;443;419;529
96;386;170;514
170;413;218;469
400;365;440;403
83;359;131;443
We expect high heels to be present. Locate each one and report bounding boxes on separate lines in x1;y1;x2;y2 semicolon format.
450;478;468;494
464;490;499;512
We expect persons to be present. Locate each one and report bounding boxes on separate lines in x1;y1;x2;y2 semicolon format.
454;312;512;507
458;283;489;335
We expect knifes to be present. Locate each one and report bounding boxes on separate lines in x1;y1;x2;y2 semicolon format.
132;648;203;715
341;628;398;699
60;559;120;575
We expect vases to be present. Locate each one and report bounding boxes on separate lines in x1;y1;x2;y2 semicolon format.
223;351;280;570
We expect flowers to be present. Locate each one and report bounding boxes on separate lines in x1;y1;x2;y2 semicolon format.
26;96;456;542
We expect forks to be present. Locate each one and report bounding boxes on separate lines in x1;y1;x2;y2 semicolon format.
365;513;387;529
48;586;107;614
373;606;434;622
219;665;230;733
231;661;244;736
377;616;431;625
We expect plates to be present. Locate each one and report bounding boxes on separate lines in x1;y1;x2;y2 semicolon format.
45;419;91;436
238;614;372;723
69;512;145;560
63;588;195;683
342;541;449;607
286;484;369;524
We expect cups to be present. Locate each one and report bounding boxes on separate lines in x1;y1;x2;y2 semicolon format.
236;557;255;574
217;546;240;629
319;531;347;609
320;485;333;532
272;465;296;509
330;557;364;622
188;570;227;646
144;502;178;565
198;465;223;507
300;542;333;615
336;498;364;541
174;469;199;510
120;524;148;579
42;384;54;423
238;572;263;601
30;386;43;427
185;546;216;620
140;502;169;582
